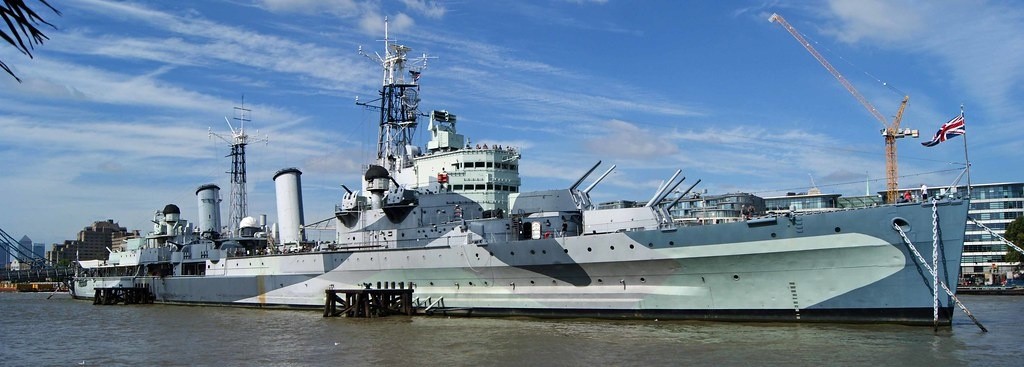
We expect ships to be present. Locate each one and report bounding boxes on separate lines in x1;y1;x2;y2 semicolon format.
597;182;1024;296
67;16;971;329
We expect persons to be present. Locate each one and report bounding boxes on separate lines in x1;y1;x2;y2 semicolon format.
256;247;260;255
562;221;568;233
276;246;287;253
697;217;701;223
546;219;551;227
741;203;755;215
482;144;488;149
905;190;912;198
476;144;481;149
920;184;928;202
492;144;502;150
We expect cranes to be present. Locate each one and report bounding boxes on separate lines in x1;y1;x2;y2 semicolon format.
768;12;919;204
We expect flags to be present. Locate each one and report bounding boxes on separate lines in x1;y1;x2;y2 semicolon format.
409;71;420;81
921;113;966;147
